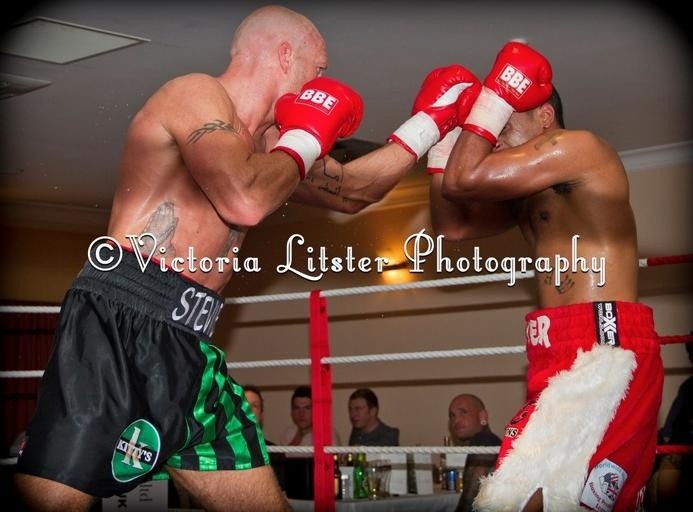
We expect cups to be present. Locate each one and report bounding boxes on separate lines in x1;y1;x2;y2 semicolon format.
367;458;391;501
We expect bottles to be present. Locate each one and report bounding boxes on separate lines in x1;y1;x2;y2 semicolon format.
331;449;369;501
439;434;451;492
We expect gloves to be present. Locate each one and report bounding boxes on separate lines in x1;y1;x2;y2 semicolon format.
462;42;554;147
270;76;364;182
386;64;483;174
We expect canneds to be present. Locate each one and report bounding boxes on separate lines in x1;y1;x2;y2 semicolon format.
448;469;459;491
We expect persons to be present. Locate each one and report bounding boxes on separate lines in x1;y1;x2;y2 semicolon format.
347;388;400;446
279;386;342;445
653;331;693;512
448;394;502;512
240;384;275;445
426;40;665;512
0;4;482;512
